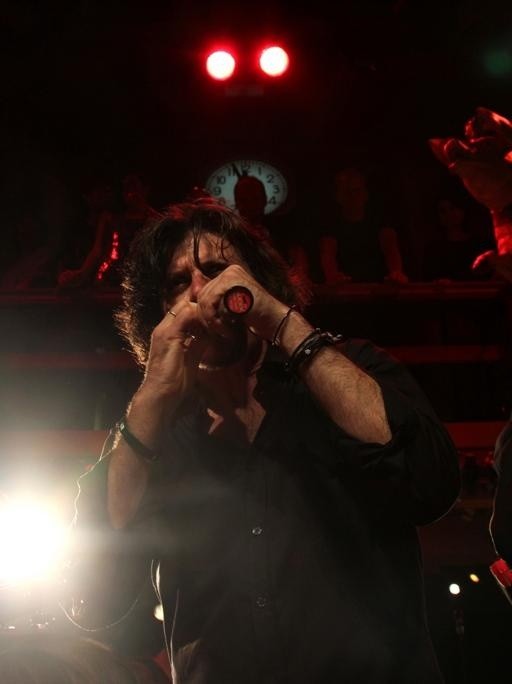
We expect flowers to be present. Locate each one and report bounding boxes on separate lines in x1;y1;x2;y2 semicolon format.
216;285;254;319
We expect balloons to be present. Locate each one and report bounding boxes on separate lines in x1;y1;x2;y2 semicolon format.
197;156;295;219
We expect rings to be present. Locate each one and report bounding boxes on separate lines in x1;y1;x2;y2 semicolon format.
167;311;176;317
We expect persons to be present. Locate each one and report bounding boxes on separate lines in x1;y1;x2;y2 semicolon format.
440;184;510;421
57;163;158;287
58;199;460;684
319;167;410;346
234;176;309;275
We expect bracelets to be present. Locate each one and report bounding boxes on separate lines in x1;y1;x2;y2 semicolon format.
284;328;341;378
117;417;159;461
272;305;296;347
